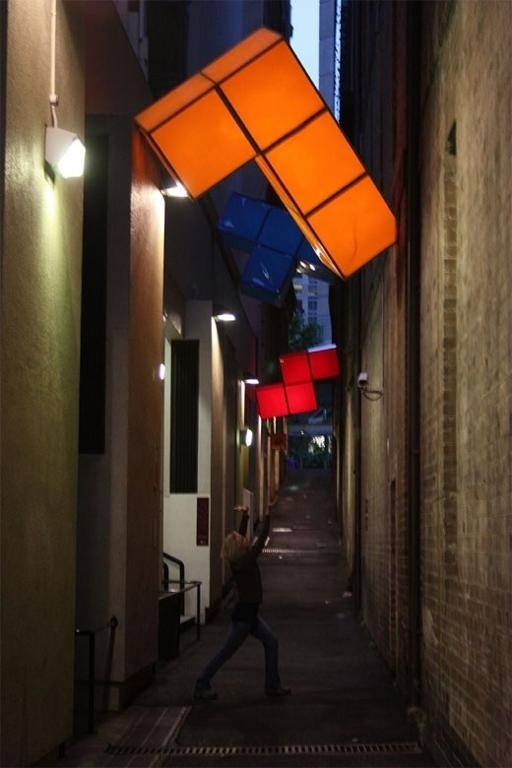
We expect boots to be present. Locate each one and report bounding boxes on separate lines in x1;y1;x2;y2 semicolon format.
195;678;218;700
265;676;291;696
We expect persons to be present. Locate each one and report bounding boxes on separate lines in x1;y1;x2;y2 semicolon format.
191;495;293;701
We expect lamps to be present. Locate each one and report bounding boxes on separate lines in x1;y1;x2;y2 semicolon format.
44;125;88;180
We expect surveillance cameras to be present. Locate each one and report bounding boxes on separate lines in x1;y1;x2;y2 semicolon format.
356;372;369;390
